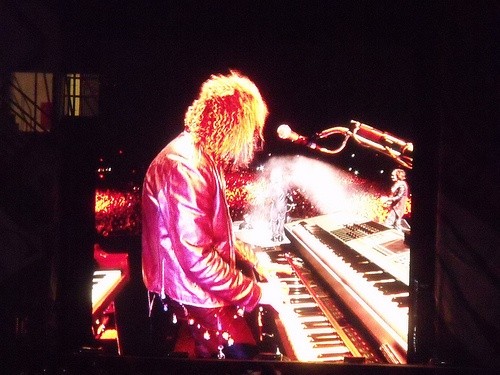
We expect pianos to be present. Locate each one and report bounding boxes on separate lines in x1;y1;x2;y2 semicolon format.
245;208;411;366
93;246;131;325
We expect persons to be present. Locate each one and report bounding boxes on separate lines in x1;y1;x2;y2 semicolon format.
140;71;291;363
383;167;408;234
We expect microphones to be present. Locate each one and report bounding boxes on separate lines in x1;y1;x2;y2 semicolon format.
276;124;321;151
